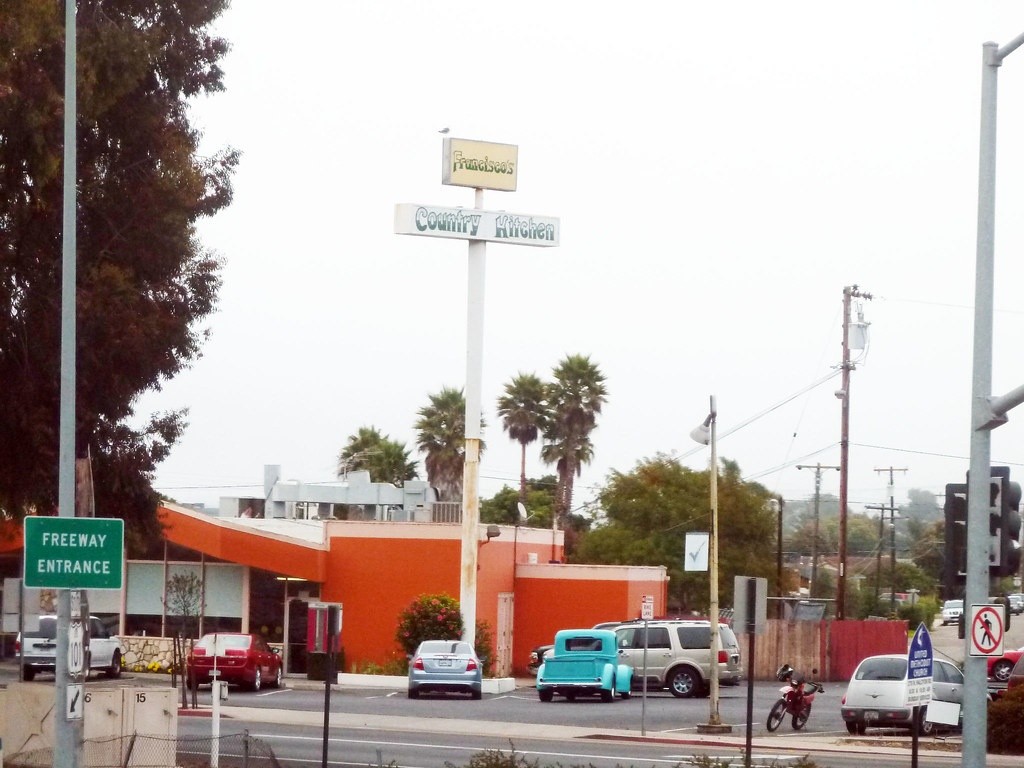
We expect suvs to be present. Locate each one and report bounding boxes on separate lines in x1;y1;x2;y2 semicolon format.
527;620;633;677
541;617;742;699
14;614;126;681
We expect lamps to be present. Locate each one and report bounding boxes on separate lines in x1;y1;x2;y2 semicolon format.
478;525;501;547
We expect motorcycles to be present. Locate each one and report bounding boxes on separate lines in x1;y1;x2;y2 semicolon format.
765;664;825;733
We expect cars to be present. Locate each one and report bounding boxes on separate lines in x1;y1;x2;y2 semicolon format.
408;639;488;700
986;649;1024;682
185;632;284;692
535;628;634;704
841;654;995;736
942;598;965;625
879;593;912;608
1007;593;1024;615
1007;653;1024;692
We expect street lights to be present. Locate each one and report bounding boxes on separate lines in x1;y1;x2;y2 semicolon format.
688;394;735;732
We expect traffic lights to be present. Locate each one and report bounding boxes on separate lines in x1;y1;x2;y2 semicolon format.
983;464;1022;576
